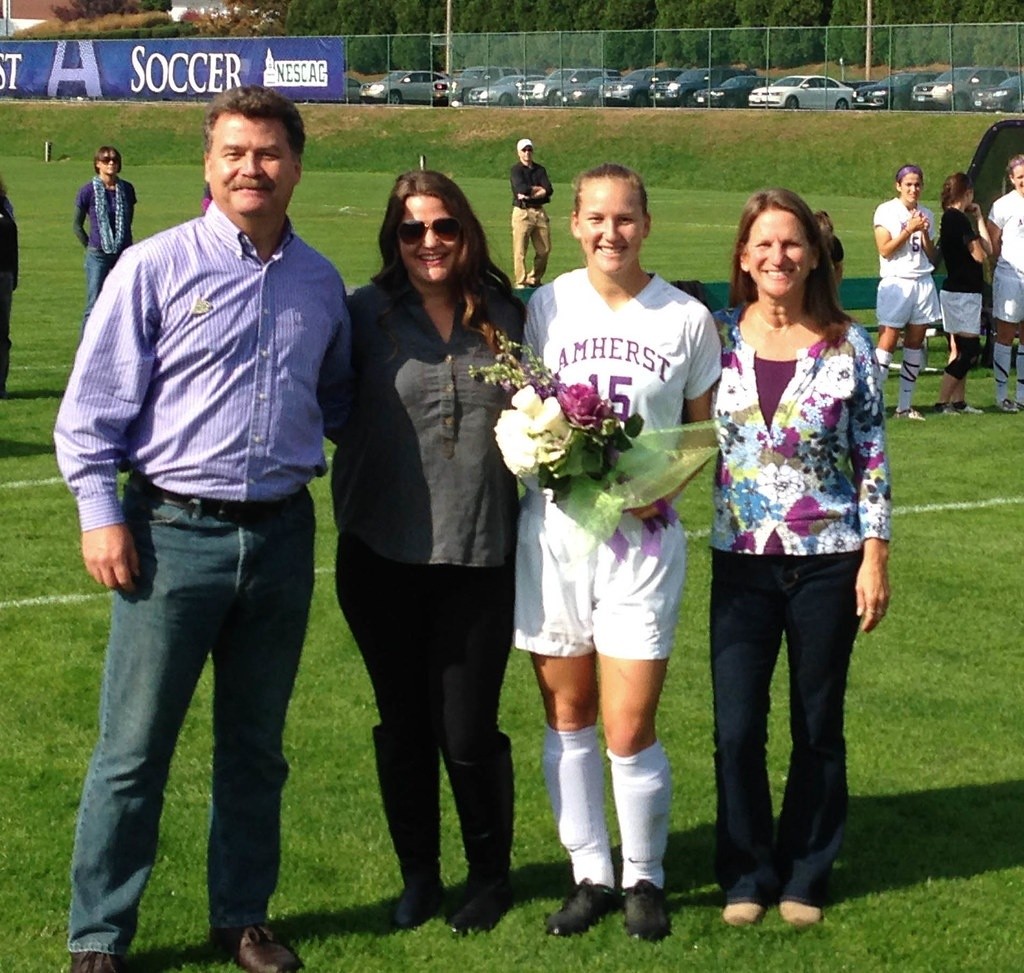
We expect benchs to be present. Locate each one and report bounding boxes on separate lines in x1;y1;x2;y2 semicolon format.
509;275;989;383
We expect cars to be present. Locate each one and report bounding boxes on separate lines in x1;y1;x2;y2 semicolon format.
693;76;776;109
748;74;856;111
852;71;944;112
558;76;624;107
307;76;364;104
469;74;545;107
971;75;1024;114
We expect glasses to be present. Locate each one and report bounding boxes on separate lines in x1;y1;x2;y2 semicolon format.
98;156;118;164
521;148;533;152
395;217;461;245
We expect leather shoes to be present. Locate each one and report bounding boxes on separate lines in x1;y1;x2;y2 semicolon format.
70;952;123;973
208;921;303;973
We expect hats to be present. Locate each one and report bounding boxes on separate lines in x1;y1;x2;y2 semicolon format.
516;139;533;151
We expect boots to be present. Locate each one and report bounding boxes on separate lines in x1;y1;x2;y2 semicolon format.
444;730;513;930
373;723;443;931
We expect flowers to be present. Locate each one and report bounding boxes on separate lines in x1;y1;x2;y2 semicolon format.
471;332;677;535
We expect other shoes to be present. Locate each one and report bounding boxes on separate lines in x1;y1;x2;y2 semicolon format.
894;407;925;420
950;401;983;414
1013;400;1024;410
779;901;821;925
996;399;1019;412
526;282;542;288
934;402;960;414
723;902;767;927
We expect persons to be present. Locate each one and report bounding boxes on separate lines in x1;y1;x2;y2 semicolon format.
987;153;1024;412
933;170;993;415
315;170;526;935
814;209;844;285
874;164;941;419
0;174;18;398
53;84;353;973
521;163;722;941
73;147;138;341
712;188;893;927
510;139;554;288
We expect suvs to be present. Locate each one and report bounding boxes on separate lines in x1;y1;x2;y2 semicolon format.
359;70;454;107
647;66;759;110
911;66;1019;112
432;66;523;107
517;68;622;109
598;68;687;108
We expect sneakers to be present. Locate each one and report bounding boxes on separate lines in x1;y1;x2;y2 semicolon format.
618;879;674;942
545;879;620;937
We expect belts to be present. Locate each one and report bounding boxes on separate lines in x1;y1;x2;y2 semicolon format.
127;472;307;520
